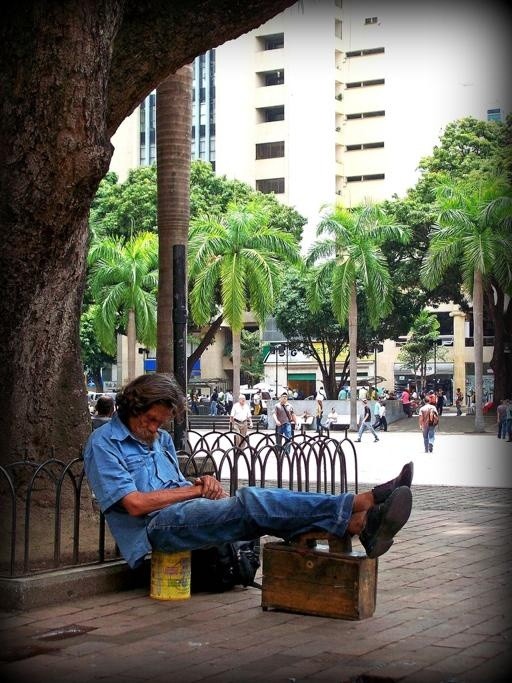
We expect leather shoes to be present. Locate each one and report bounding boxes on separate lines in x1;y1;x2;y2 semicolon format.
373;463;413;504
358;486;413;559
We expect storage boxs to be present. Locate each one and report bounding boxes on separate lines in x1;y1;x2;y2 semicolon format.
259;534;381;624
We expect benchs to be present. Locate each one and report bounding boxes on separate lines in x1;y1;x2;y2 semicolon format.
327;412;366;427
290;415;314;429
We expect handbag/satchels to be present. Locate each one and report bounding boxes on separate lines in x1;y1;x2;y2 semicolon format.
192;540;257;594
428;408;439;427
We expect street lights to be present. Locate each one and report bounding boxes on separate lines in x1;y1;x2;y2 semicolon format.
432;340;439;399
269;345;285;398
138;347;150;376
371;338;380;399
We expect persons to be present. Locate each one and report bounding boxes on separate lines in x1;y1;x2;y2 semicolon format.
229;394;253;454
273;392;296;457
186;385;511;442
81;369;417;571
418;395;439;454
91;395;115;427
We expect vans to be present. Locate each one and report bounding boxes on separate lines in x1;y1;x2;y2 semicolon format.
240;389;273;414
90;393;116;408
